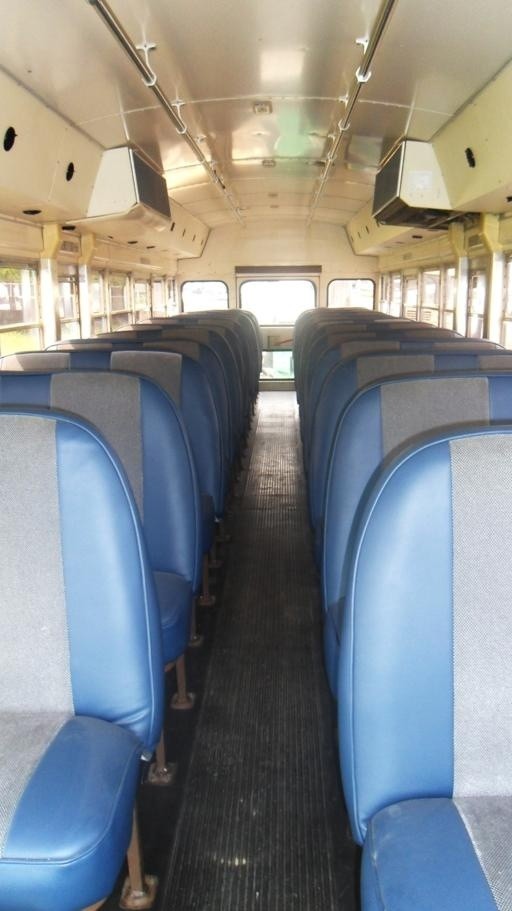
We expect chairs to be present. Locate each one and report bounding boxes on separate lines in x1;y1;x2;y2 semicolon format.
290;307;511;911
0;305;262;907
